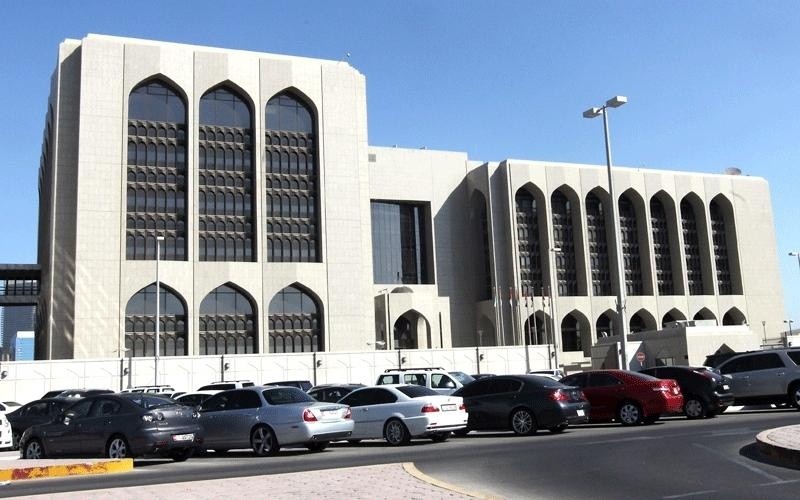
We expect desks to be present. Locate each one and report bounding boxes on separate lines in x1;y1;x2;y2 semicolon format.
761;320;769;343
789;251;800;265
154;236;165;388
582;94;632;371
784;319;795;336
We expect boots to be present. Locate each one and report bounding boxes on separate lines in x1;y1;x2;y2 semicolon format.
712;347;799;411
375;364;474;396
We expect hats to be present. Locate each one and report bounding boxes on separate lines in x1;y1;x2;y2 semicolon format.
448;366;735;436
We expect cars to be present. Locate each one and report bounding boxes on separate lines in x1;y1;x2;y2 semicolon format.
19;392;203;463
1;381;254;453
193;385;353;458
448;366;735;436
334;383;467;447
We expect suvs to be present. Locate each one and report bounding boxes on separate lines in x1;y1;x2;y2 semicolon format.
712;347;799;411
265;381;314;394
375;364;474;396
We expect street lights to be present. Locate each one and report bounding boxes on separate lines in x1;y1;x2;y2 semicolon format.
582;94;632;371
784;319;795;336
789;251;800;265
761;320;769;343
154;236;165;388
